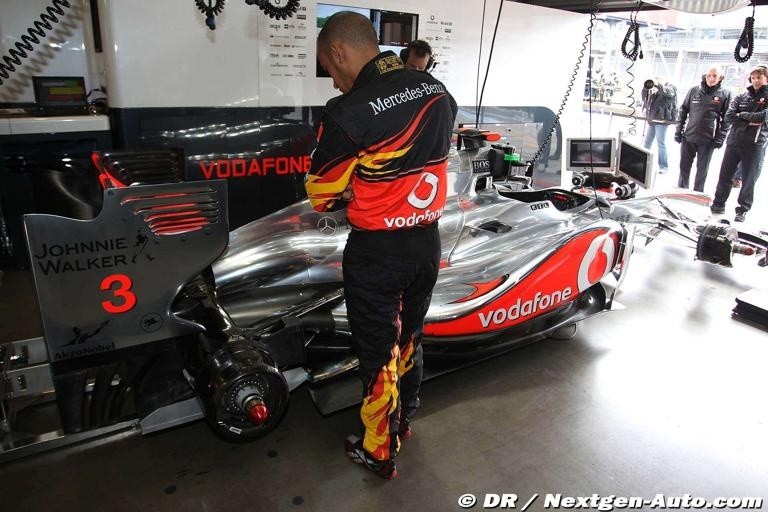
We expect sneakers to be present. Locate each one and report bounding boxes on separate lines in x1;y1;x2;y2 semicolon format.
710;203;725;214
732;179;742;188
344;417;413;480
734;209;746;222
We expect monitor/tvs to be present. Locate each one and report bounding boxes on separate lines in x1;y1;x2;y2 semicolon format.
618;138;653;190
565;138;616;173
31;75;88;111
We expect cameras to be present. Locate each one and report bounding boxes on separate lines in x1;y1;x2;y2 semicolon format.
643;77;656;91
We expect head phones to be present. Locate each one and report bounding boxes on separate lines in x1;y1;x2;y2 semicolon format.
702;74;724;84
748;66;768;84
399;48;435;71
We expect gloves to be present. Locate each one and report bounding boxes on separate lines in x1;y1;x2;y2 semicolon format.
674;132;682;143
713;137;724;148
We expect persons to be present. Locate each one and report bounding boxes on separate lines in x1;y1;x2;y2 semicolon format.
398;38;437;74
640;70;678;176
673;65;732;194
730;160;744;190
700;65;767;223
300;7;462;481
